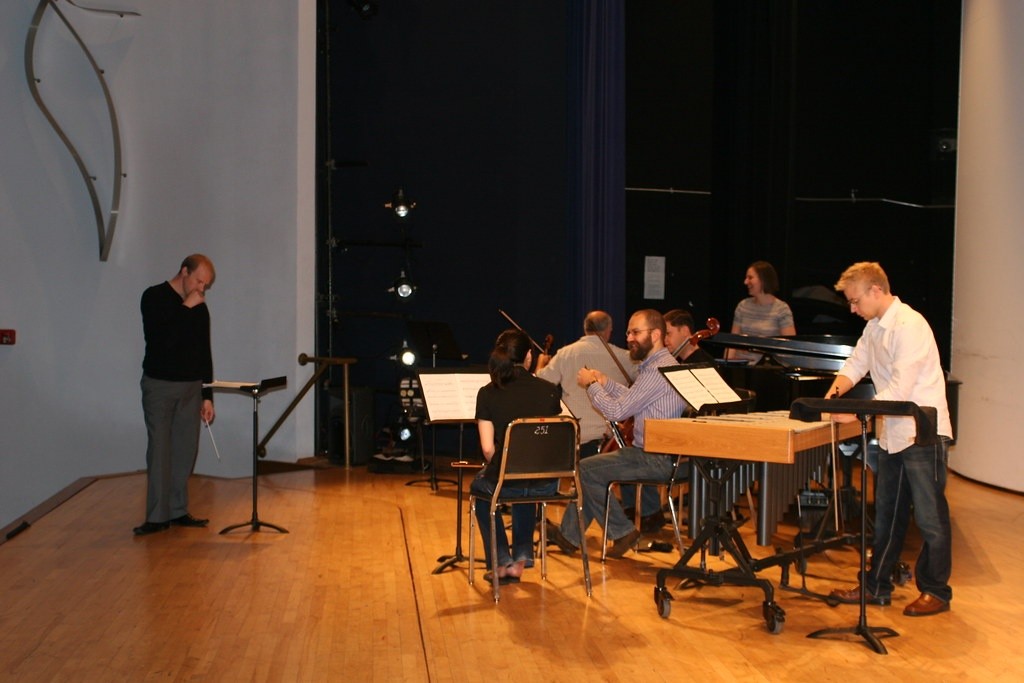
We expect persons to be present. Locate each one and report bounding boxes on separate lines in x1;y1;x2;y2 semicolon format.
535;311;643;460
475;332;562;583
538;310;687;557
723;261;796;366
823;262;954;616
133;253;216;536
620;310;717;533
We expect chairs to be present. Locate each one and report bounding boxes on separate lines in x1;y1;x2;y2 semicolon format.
470;416;592;604
601;406;696;564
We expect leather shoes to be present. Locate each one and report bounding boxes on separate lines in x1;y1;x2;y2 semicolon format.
903;591;949;616
830;583;891;605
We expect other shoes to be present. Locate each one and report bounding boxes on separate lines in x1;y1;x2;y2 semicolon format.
171;512;210;526
133;520;171;535
542;519;579;559
622;507;667;534
605;527;642;560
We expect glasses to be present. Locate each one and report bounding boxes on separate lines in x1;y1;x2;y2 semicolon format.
626;327;657;336
844;286;878;306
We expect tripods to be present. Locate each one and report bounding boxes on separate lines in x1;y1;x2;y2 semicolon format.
404;352;459;491
786;397;937;656
416;368;493;575
202;375;290;535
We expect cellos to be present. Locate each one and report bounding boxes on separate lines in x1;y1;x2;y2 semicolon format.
600;317;720;454
544;334;553;355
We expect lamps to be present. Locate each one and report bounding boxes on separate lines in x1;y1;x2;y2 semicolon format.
384;188;417;218
388;270;418;299
389;339;417;368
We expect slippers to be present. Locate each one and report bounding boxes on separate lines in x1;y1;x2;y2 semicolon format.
508;573;520;583
484;571;507;585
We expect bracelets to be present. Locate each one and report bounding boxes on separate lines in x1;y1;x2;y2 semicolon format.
586;380;597;388
